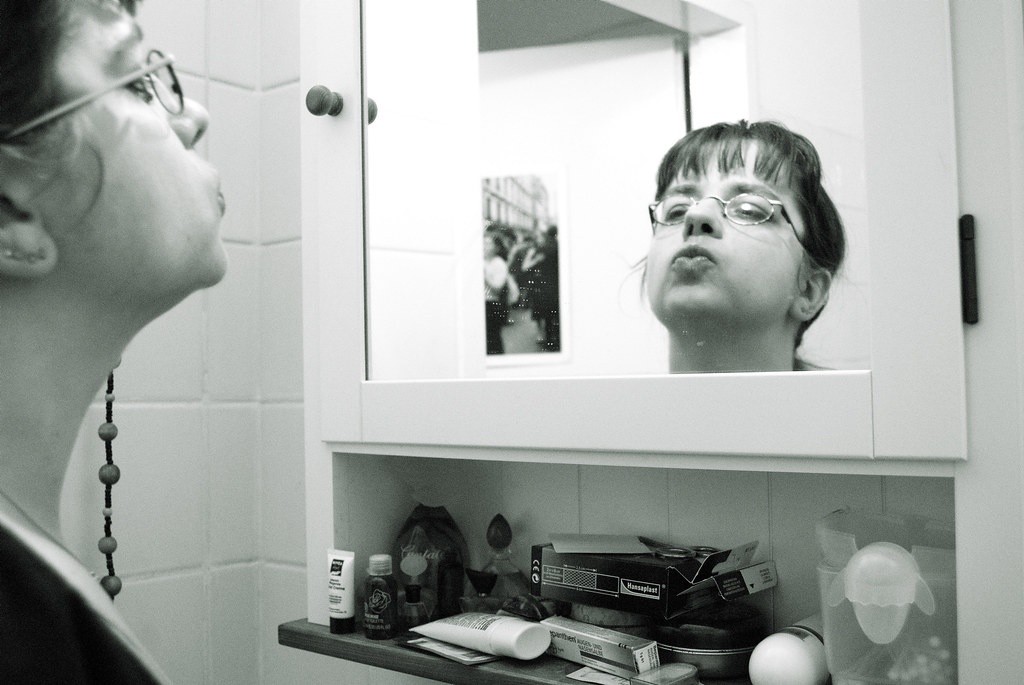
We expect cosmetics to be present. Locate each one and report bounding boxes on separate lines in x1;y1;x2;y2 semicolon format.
326;548;430;639
409;611;551;659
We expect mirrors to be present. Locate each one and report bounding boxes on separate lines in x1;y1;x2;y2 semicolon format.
361;0;867;380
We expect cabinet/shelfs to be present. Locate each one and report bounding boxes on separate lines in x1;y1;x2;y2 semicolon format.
277;0;1024;685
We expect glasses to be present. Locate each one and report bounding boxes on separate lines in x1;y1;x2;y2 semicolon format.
649;193;817;256
0;49;184;143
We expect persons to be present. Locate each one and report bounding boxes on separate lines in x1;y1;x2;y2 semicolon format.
483;221;560;355
618;119;846;374
0;0;230;685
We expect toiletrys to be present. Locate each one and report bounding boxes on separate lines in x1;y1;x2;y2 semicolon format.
407;611;550;660
327;549;356;632
